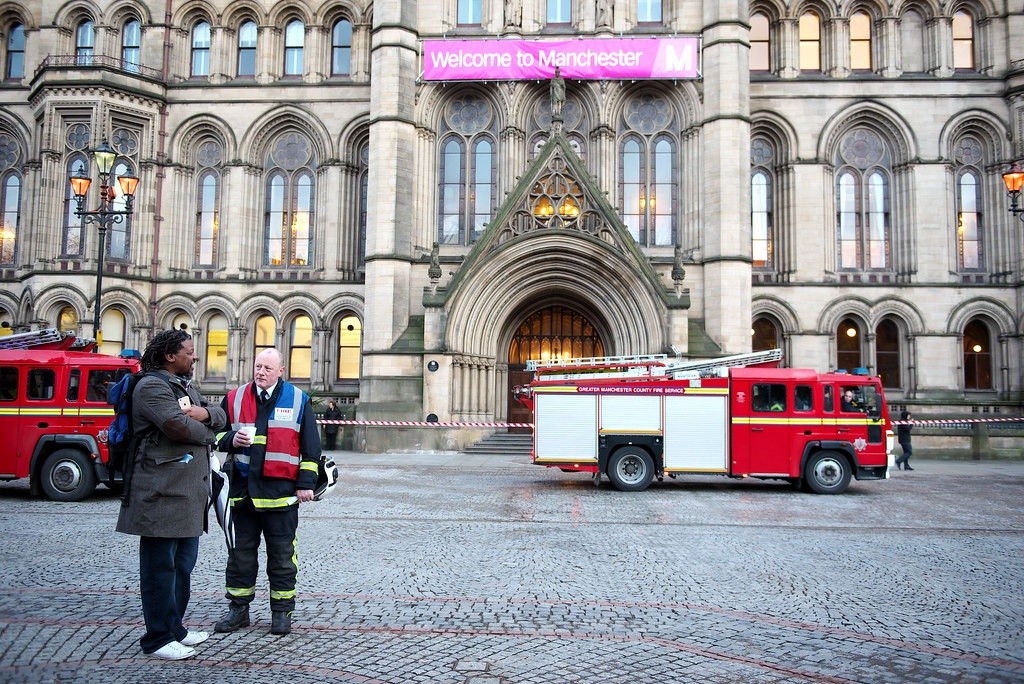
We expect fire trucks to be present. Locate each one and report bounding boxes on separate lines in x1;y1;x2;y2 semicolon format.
517;348;895;495
0;328;142;503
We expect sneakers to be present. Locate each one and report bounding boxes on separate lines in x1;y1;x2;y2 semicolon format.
180;630;210;646
148;641;195;661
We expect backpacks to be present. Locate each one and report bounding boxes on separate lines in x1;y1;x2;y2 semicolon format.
106;369;175;508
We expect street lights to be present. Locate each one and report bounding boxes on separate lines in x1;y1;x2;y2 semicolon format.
69;137;140;342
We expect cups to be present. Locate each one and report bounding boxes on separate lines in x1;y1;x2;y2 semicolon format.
241;426;257;445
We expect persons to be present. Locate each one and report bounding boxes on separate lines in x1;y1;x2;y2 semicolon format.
840;390;861;413
88;371;111;402
211;347;324;634
895;411;915;470
115;328;226;660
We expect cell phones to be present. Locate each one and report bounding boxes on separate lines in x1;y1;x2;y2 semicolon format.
179;396;191;410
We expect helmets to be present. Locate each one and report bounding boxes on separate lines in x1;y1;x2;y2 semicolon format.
312;455;338;501
102;373;111;382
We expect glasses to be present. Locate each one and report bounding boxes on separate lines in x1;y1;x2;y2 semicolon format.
844;395;851;398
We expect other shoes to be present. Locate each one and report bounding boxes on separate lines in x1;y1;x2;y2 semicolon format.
214;601;250;632
895;460;900;469
904;467;914;470
271;611;291;634
